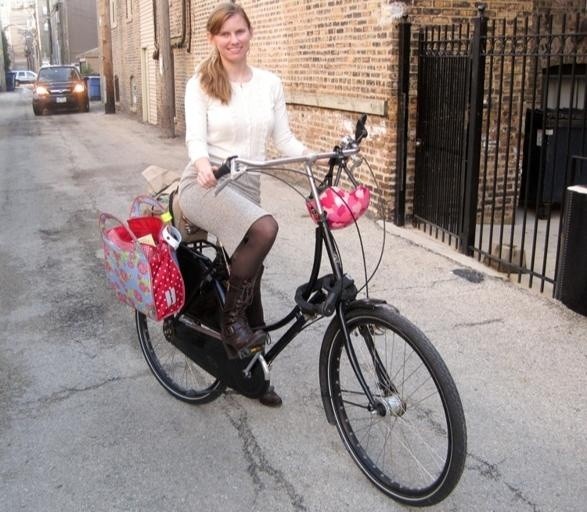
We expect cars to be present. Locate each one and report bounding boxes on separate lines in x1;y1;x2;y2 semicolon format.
32;65;89;115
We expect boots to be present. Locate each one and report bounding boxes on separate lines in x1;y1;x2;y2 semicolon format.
249;319;284;408
221;270;257;350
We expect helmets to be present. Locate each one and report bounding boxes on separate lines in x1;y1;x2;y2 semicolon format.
303;181;370;231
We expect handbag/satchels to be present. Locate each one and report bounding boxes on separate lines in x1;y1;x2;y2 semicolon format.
97;193;188;324
141;164;210;242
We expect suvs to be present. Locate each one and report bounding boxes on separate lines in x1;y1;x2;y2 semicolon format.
11;71;38;87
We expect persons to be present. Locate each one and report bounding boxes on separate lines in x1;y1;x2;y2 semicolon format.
178;2;352;407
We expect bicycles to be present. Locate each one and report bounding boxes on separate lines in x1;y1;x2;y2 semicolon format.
135;137;468;507
